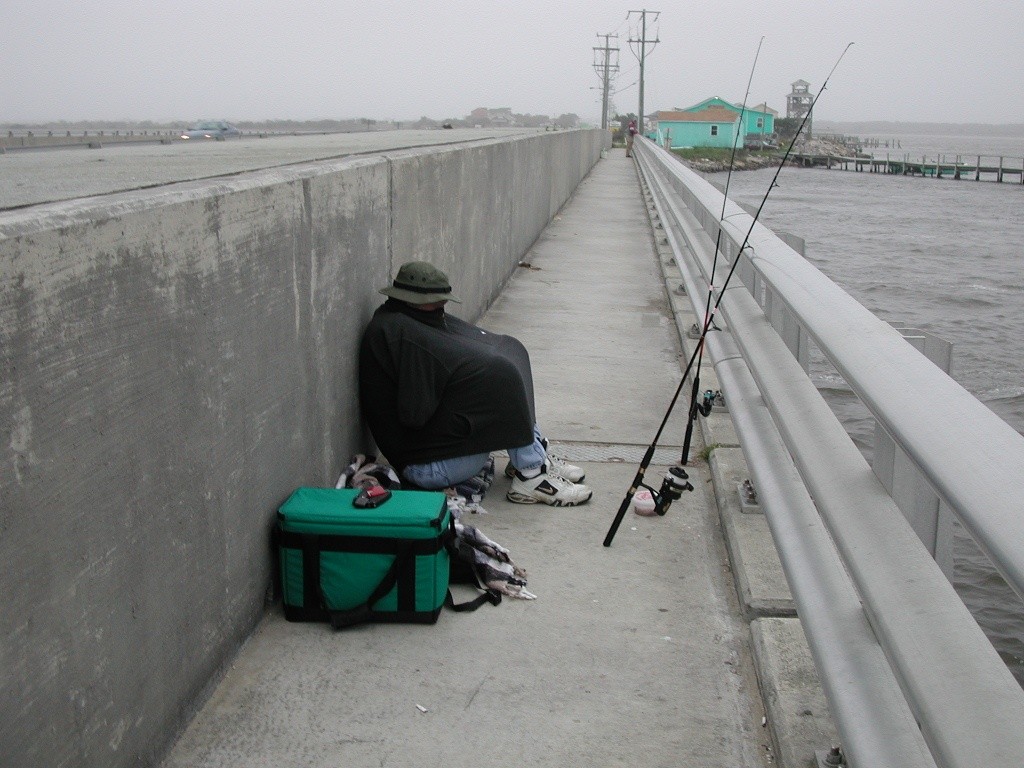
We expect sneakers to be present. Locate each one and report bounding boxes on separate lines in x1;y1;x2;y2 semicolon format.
506;463;593;509
503;436;586;479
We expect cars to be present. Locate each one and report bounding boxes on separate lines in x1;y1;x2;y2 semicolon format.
180;120;239;141
744;139;779;152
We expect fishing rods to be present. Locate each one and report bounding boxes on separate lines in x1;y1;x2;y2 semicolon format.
601;32;859;551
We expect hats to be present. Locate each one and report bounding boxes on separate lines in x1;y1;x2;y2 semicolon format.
376;260;464;305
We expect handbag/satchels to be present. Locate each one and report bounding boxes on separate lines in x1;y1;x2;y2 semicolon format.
274;485;457;626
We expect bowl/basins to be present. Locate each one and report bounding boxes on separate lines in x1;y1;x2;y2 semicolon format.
632;490;658;516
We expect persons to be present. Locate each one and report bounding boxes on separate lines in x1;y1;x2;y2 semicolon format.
625;117;640;158
358;260;594;508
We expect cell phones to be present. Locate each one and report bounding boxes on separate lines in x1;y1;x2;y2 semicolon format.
367;492;392;508
352;487;370;508
